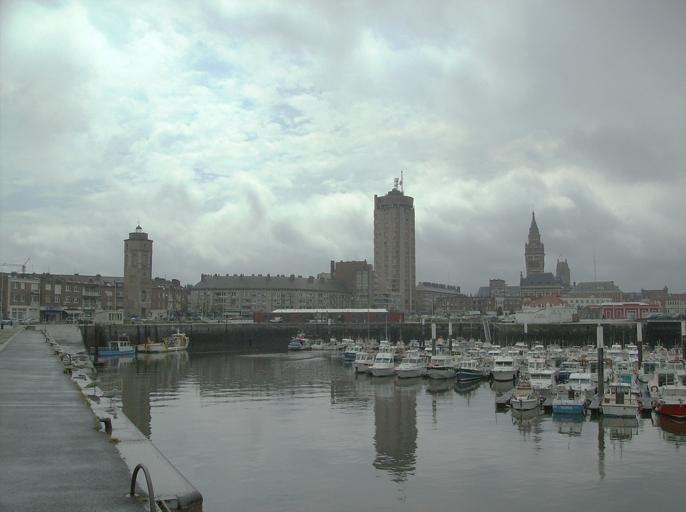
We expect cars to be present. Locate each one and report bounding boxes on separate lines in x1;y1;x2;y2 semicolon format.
419;314;517;323
634;311;686;321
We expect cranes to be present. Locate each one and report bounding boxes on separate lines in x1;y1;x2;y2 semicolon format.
0;256;31;273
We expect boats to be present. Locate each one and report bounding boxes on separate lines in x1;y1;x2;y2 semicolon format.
354;373;516;397
288;331;527;381
138;351;188;365
512;407;686;450
89;350;133;370
507;334;686;419
137;331;189;353
89;333;135;354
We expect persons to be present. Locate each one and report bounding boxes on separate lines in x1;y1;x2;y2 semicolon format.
297;330;305;346
